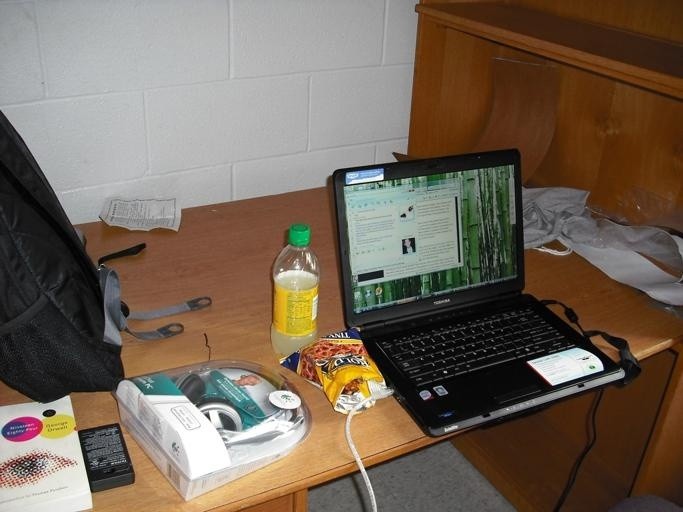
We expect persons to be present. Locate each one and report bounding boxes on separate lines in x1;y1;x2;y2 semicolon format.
403;239;415;254
232;372;261;396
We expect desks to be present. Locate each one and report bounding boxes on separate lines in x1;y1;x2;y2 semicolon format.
0;184;682;512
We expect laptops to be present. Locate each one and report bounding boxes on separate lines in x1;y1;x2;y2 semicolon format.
332;149;626;438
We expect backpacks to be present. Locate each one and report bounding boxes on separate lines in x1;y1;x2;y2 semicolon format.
0;102;129;403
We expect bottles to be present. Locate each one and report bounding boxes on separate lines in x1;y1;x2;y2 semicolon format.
269;221;320;357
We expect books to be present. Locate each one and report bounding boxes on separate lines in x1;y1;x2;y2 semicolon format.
0;396;93;511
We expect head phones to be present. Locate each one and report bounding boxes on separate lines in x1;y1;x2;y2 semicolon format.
174;366;305;449
